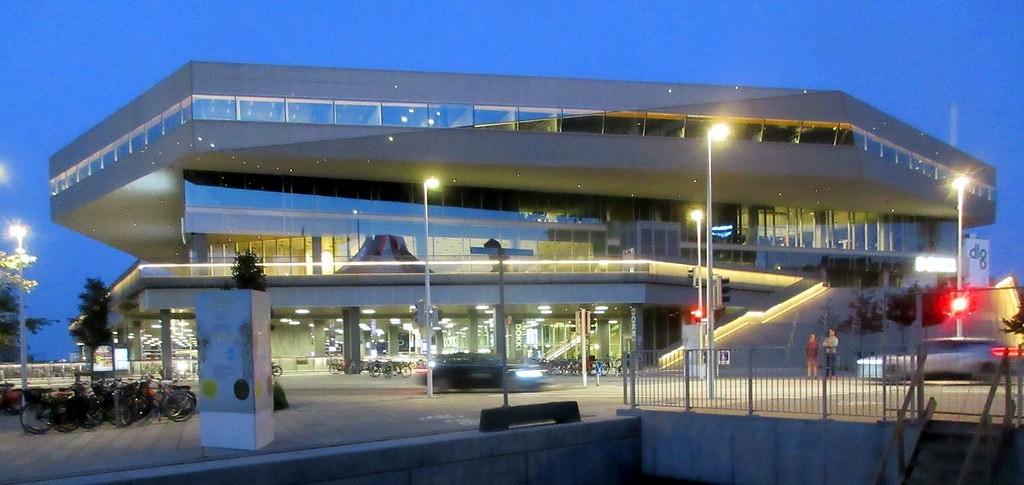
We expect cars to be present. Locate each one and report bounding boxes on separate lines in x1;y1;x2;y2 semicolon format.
424;355;544;392
883;336;1008;382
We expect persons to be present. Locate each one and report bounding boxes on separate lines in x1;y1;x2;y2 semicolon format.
804;333;821;380
823;328;839;381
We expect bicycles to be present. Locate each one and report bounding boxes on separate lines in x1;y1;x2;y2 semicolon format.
328;357;412;379
533;355;598;376
600;354;630;377
271;361;283;377
20;373;197;435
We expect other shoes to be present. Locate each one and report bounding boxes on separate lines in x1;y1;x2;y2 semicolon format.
826;376;829;379
806;377;812;380
814;376;819;380
830;376;835;379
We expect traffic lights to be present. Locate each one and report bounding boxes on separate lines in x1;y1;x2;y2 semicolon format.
716;275;730;311
688;267;697;287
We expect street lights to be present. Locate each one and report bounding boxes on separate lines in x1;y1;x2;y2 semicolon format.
8;224;28;409
423;176;441;397
691;211;705;382
706;123;730;401
951;177;969;338
